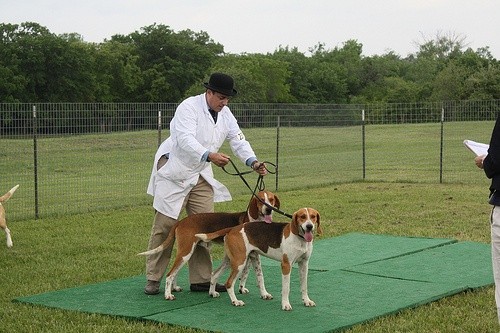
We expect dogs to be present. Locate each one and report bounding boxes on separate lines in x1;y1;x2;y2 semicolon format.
135;191;280;300
0;184;19;248
194;207;322;310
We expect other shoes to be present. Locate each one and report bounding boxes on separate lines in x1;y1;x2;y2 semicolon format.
190;282;227;292
145;280;160;295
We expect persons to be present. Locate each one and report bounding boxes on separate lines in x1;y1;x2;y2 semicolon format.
474;112;500;325
143;71;267;295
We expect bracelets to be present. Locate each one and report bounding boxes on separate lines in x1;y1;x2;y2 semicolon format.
251;161;258;168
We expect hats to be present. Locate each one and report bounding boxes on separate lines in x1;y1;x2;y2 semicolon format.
203;73;237;95
